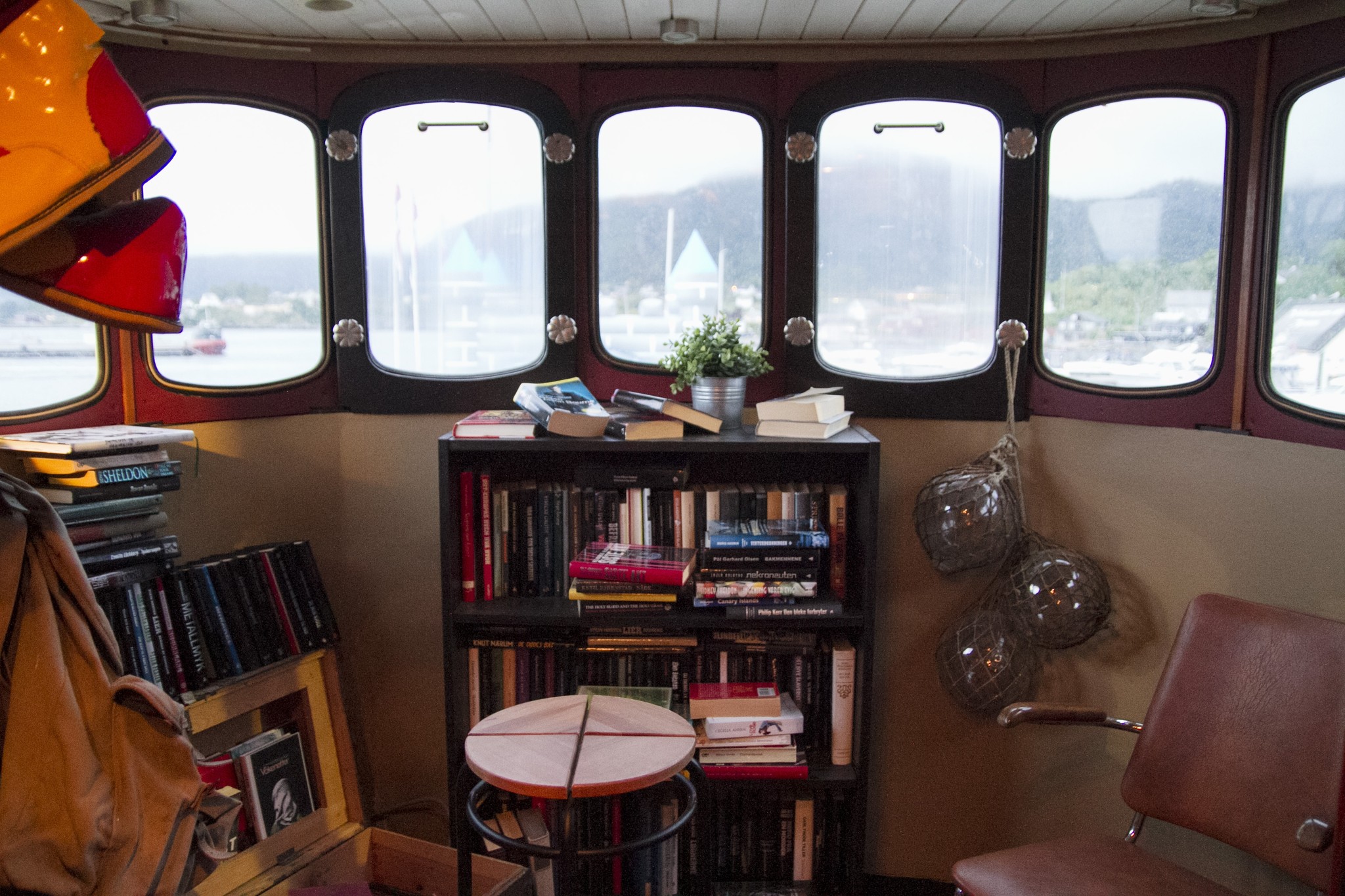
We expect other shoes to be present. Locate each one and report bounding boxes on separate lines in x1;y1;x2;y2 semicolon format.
0;0;177;258
1;196;186;334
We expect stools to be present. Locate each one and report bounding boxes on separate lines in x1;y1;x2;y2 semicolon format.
465;695;699;896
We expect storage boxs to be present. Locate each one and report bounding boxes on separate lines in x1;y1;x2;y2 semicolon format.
158;649;528;896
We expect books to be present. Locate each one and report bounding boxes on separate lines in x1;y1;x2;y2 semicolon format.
454;373;725;441
194;718;316;863
458;464;854;896
756;386;856;440
0;427;350;696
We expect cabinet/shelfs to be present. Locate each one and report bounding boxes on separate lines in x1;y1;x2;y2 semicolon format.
438;432;878;896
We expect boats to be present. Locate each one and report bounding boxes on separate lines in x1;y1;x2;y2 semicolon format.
0;311;228;357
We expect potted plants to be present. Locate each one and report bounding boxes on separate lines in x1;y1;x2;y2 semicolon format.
661;312;774;430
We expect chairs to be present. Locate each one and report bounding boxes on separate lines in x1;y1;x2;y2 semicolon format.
951;593;1345;896
